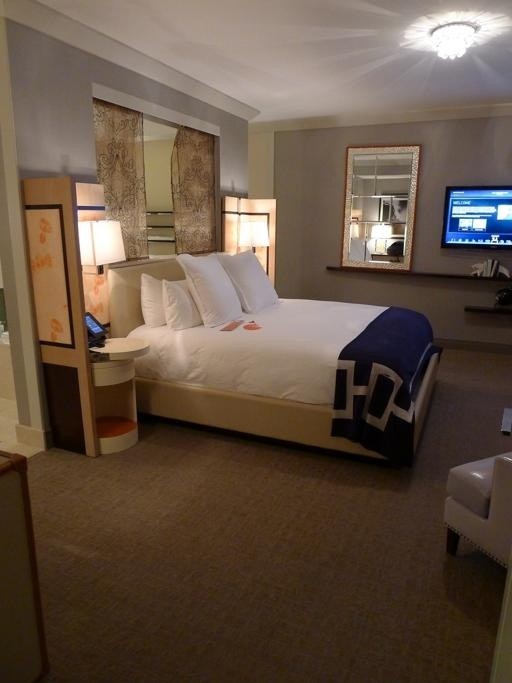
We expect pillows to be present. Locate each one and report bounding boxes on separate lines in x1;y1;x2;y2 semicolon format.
163;279;204;330
177;252;243;328
217;250;280;313
140;272;166;327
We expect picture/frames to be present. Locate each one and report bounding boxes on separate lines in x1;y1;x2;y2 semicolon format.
379;192;408;224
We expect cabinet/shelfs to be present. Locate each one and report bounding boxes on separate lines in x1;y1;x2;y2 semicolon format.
146;211;174;242
327;266;512;313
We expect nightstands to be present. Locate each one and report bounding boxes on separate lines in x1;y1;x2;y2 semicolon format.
89;338;149;454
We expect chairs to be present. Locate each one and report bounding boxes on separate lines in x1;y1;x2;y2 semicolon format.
444;453;512;568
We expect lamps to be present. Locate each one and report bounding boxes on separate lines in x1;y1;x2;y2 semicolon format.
78;219;126;276
237;222;270;253
371;224;392;252
431;23;478;59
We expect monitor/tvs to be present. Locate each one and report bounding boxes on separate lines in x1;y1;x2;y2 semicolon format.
85;312;109;338
440;185;512;250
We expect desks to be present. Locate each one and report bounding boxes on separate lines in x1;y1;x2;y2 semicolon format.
0;450;49;682
371;254;403;262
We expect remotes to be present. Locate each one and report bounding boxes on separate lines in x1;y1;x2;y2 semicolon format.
220;319;244;332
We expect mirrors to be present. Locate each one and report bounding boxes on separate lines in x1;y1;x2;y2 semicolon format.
340;146;421;269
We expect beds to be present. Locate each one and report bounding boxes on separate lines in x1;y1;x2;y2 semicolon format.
106;256;443;469
